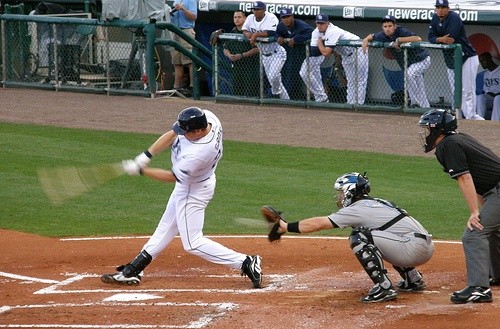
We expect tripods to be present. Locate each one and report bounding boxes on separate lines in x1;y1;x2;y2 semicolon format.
119;38;147;90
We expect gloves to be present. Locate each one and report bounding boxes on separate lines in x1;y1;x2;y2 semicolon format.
123;149;153;176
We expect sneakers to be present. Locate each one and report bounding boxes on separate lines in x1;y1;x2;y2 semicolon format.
361;284;397;302
451;286;492;304
241;254;262;288
101;264;144;286
396;280;425;292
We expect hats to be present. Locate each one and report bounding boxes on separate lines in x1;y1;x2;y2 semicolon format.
280;9;292;18
251;1;266;9
435;0;449;7
315;14;329;23
381;15;396;23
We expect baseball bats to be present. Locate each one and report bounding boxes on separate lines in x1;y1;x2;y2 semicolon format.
36;161;134;210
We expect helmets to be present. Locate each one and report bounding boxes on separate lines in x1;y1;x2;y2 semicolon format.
418;109;457;153
172;107;207;135
334;172;371;208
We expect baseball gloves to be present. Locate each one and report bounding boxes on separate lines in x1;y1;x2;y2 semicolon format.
260;205;286;241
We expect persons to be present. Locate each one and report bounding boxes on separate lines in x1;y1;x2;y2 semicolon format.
427;0;481;121
171;0;197;96
223;9;260;98
275;8;314;100
242;3;291;99
416;108;500;303
361;14;431;108
310;13;369;104
261;171;435;304
476;52;500;120
101;107;262;289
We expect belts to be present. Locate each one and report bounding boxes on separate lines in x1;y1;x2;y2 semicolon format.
414;233;430;238
263;50;277;56
